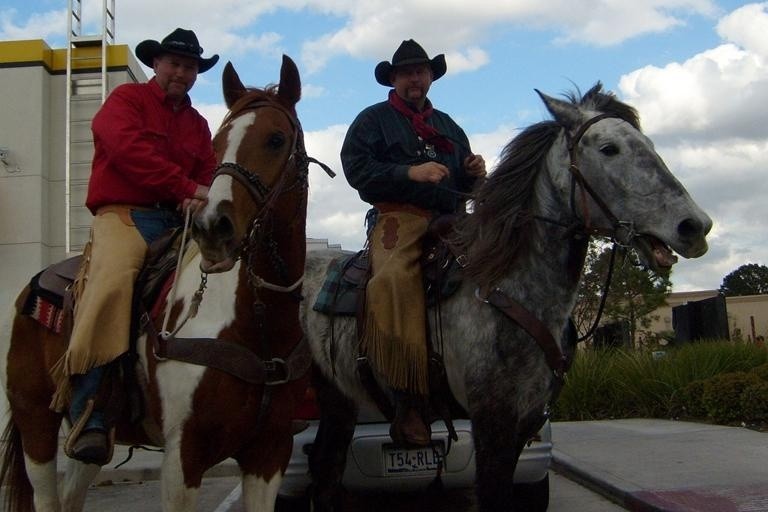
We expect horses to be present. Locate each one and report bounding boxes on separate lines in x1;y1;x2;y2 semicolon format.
296;77;714;511
0;53;309;511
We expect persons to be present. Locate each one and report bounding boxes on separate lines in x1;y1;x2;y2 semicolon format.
50;27;218;460
340;38;486;444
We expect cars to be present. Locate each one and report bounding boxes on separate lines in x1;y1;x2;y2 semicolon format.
278;420;553;512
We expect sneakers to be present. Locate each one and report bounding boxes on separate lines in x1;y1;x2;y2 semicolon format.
390;405;429;446
69;409;111;465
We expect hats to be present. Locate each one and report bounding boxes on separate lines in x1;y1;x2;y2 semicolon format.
375;39;446;86
136;28;219;73
